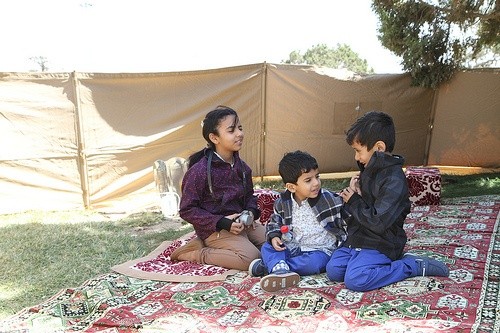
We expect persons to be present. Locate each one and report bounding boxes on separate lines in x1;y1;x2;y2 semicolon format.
170;106;267;271
248;150;361;292
326;112;449;292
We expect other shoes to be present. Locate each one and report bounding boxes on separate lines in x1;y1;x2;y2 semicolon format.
170;237;204;261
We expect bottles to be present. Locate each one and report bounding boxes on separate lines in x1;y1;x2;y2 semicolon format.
280;226;301;257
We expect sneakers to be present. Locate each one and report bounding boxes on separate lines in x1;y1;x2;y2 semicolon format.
260;269;301;292
248;259;269;277
401;252;449;277
